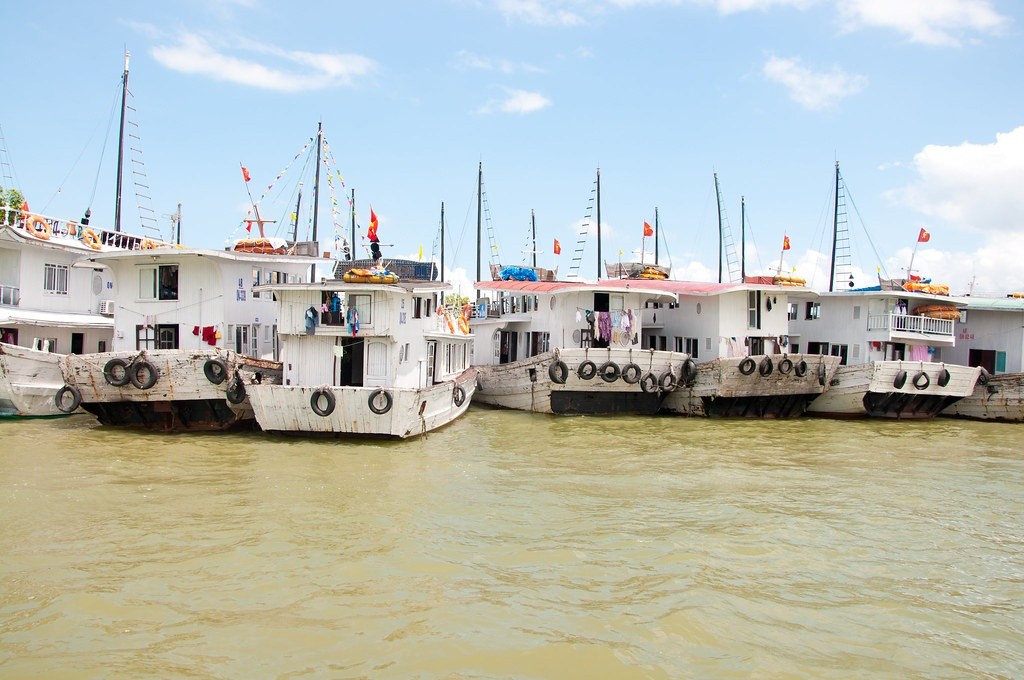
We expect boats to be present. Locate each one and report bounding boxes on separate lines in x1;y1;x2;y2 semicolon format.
59;117;356;432
930;275;1024;425
237;155;477;439
602;167;843;419
801;150;983;418
448;159;694;418
0;43;209;420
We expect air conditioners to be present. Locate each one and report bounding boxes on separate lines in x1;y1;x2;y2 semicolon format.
101;300;114;314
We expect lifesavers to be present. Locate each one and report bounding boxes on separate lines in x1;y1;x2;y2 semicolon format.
343;269;399;284
578;361;596;380
549;361;568;384
641;359;696;394
310;389;336;417
903;282;949;294
204;360;246;404
26;216;156;250
600;362;641;384
368;388;393;415
453;386;465;407
893;366;990;390
437;304;472;335
740;357;827;386
913;304;961;319
639;268;665;279
234;238;288;255
477;374;483;391
773;275;806;287
55;385;81;412
104;359;158;389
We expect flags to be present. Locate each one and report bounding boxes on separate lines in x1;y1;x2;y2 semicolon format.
367;209;380;242
918;228;930;242
783;234;790;250
644;222;653;236
910;274;921;281
554;240;561;254
242;167;251;182
18;203;31;228
246;221;252;232
